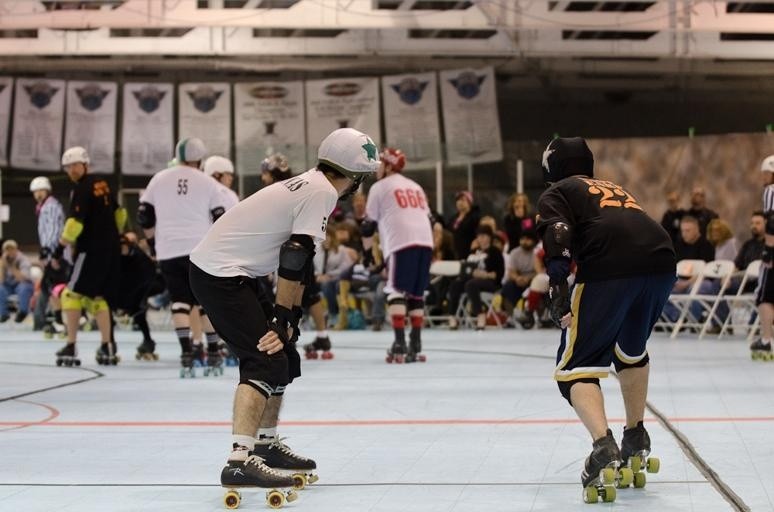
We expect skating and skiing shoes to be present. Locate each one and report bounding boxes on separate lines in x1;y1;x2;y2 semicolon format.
386;339;426;363
750;337;774;361
136;339;160;361
221;439;319;510
55;341;81;367
303;336;334;359
581;420;660;504
96;341;121;365
179;342;240;379
44;314;92;339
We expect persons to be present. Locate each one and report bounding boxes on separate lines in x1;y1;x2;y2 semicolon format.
532;135;679;488
188;125;381;490
1;136;774;369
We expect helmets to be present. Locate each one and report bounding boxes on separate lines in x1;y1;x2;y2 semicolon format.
29;176;52;192
542;136;594;186
761;155;774;173
261;153;291;181
317;128;405;183
175;137;234;176
61;146;90;165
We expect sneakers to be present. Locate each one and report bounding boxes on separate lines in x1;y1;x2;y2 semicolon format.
14;309;28;322
448;312;487;330
329;310;383;331
0;314;10;323
